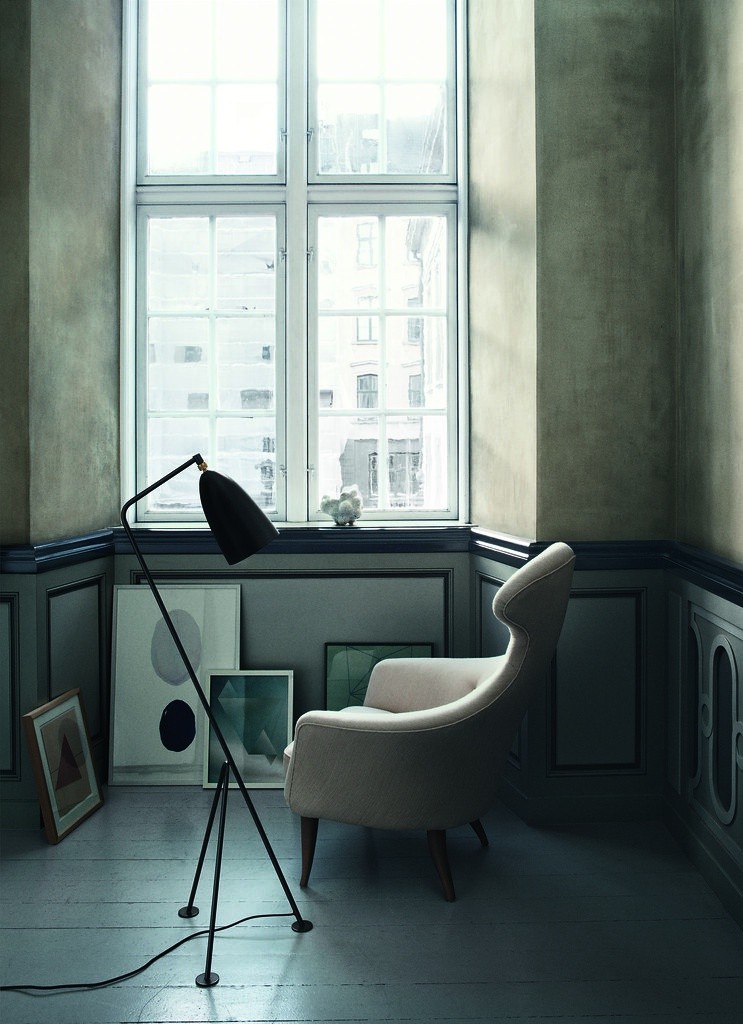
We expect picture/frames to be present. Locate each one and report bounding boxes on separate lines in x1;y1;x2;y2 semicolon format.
21;687;105;845
108;584;240;786
203;669;294;789
323;641;434;713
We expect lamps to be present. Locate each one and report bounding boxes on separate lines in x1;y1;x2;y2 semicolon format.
120;452;315;989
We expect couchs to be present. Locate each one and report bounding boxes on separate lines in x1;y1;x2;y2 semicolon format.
281;543;577;902
